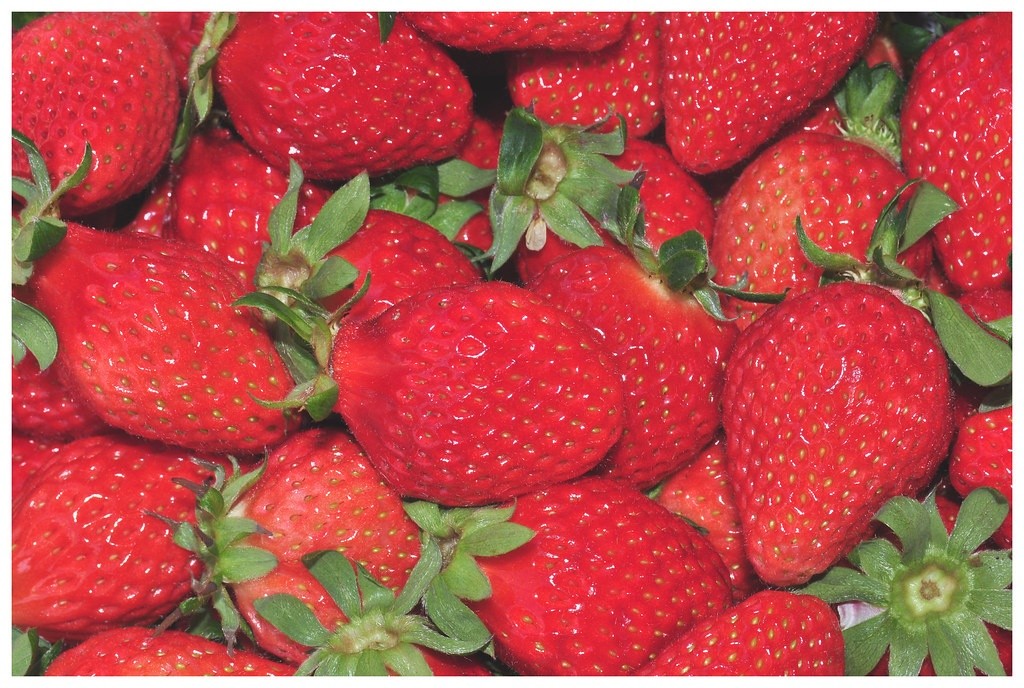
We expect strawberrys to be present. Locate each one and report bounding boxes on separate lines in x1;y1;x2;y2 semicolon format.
12;11;1012;678
